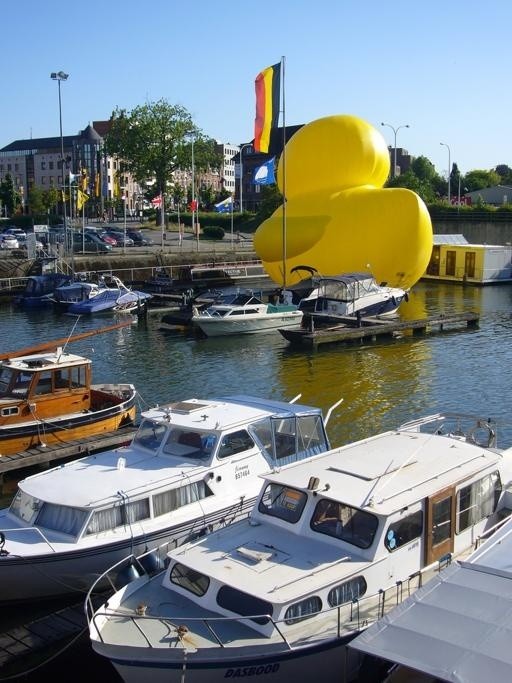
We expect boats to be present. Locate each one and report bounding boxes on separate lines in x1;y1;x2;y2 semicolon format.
84;410;512;683
10;265;304;336
0;393;347;602
0;322;141;459
295;321;348;333
297;272;410;322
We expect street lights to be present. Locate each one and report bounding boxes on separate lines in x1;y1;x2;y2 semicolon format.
50;70;69;255
381;123;410;178
440;143;451;202
185;127;202;235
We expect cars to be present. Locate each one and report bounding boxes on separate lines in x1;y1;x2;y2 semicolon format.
0;225;152;253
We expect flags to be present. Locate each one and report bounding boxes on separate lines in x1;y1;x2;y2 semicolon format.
253;61;282;155
76;188;90;212
213;195;233;214
111;169;123;197
91;173;100;198
189;196;198;213
248;155;276;186
79;168;89;190
150;195;164;210
